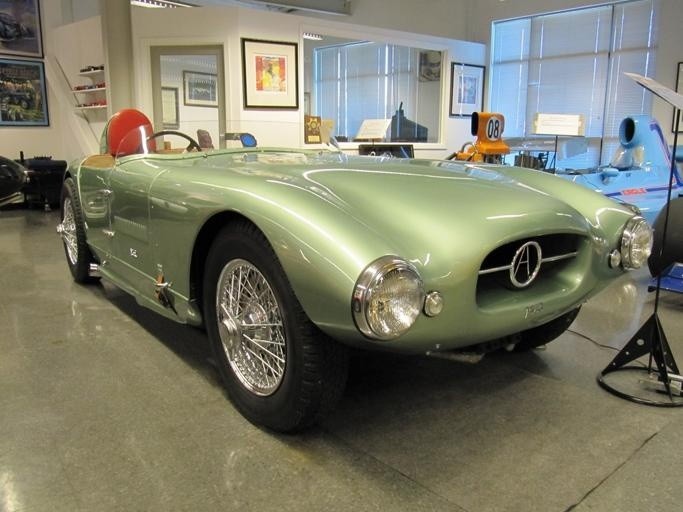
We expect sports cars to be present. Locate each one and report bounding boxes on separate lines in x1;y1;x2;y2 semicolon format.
55;109;654;435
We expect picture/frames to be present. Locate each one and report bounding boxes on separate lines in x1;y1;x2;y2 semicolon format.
161;70;217;129
0;0;49;126
449;61;486;117
669;62;683;133
239;37;299;110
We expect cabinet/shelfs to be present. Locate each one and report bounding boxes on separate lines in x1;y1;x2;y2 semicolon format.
69;69;106;110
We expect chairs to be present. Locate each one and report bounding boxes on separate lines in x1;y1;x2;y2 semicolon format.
105;110;157;160
